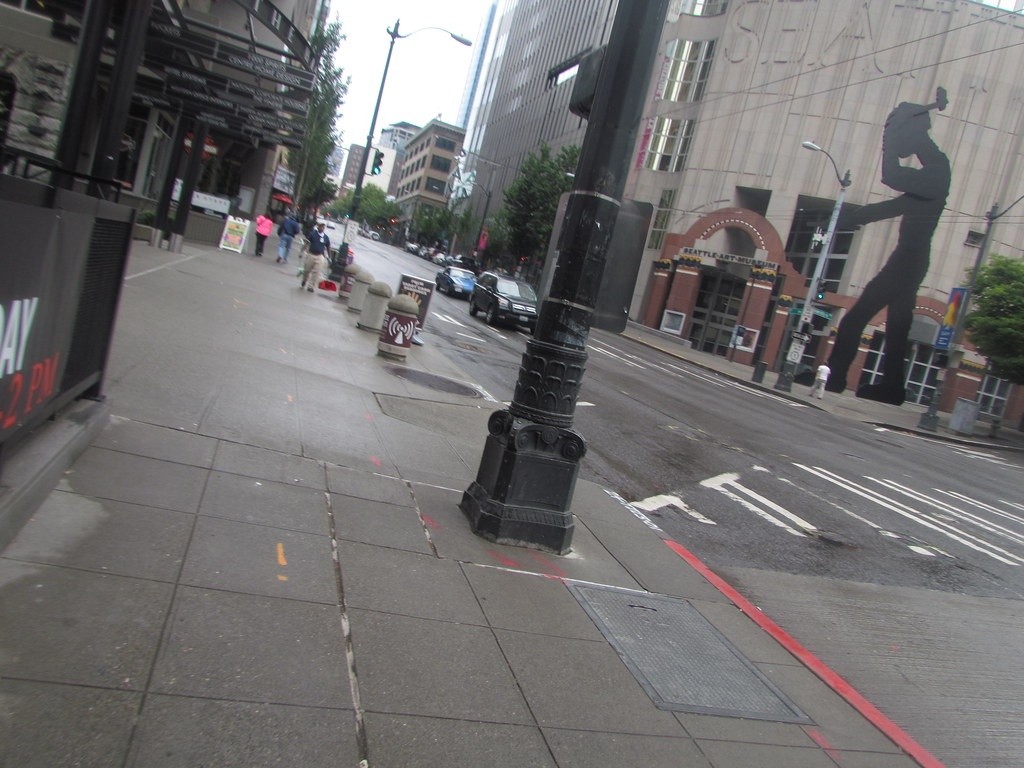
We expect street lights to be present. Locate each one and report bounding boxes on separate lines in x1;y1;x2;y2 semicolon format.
328;19;472;283
432;185;450;243
469;181;492;269
774;141;852;393
404;189;420;242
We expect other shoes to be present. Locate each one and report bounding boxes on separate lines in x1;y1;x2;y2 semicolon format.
301;280;306;287
256;251;263;257
308;287;315;293
277;256;282;262
283;259;287;264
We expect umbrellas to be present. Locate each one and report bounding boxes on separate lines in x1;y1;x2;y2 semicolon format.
273;192;293;210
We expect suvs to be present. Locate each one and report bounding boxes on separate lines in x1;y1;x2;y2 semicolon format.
469;270;537;335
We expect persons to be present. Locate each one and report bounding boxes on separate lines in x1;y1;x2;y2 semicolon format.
277;213;300;263
298;222;332;292
810;362;831;399
255;212;273;256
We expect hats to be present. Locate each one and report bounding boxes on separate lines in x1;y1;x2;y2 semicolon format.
317;220;327;226
289;213;296;221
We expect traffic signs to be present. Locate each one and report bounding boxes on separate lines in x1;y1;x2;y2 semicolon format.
791;330;813;344
788;307;804;315
810;305;835;321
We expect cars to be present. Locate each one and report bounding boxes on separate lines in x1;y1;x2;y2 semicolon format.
435;266;476;301
358;226;380;240
406;242;480;277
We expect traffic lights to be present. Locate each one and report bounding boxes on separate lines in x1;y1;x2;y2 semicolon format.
815;279;828;301
371;150;384;175
